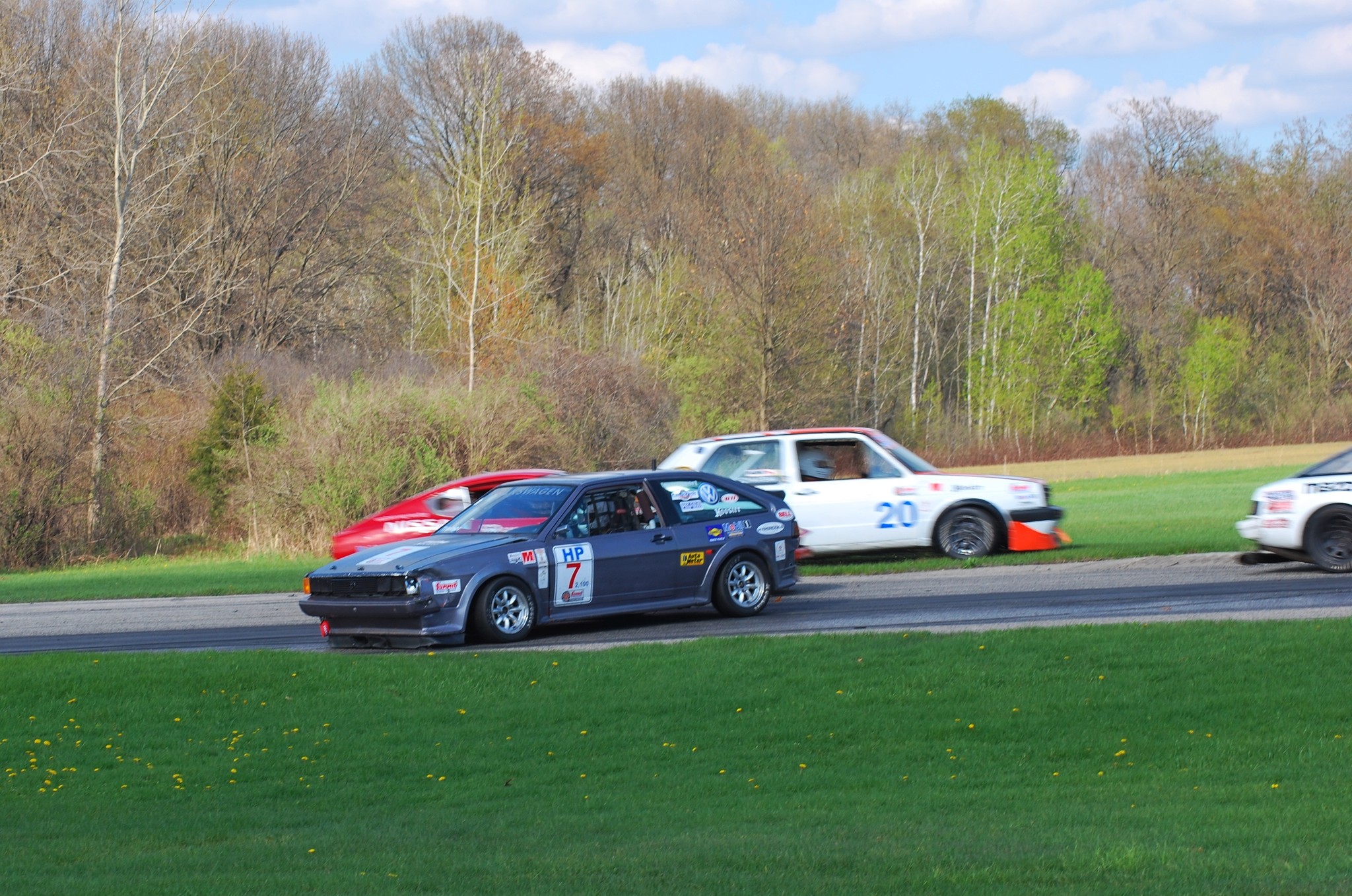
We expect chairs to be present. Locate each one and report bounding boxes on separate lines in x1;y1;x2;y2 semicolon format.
608;496;640;531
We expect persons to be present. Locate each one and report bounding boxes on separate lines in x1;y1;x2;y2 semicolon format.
799;448;835;481
556;491;625;539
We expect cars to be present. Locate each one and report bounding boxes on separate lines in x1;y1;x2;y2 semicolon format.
1233;445;1352;576
630;425;1067;565
296;464;803;647
328;467;651;562
